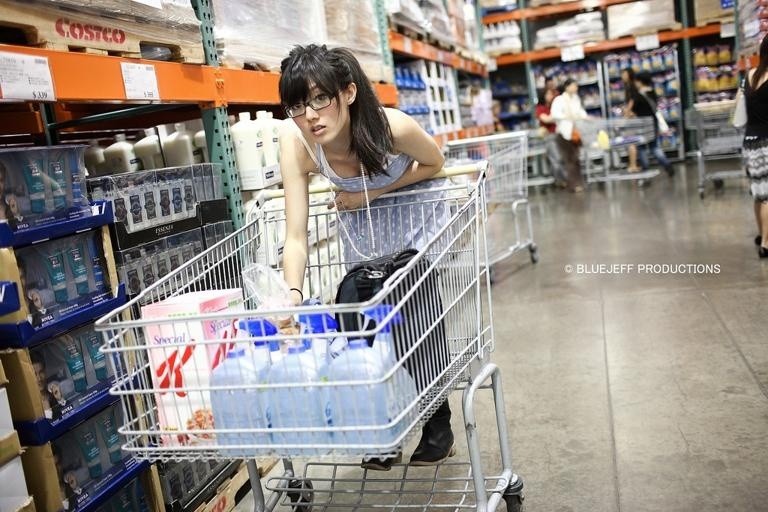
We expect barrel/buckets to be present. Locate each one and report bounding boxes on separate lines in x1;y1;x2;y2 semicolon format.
207;338;406;459
84;109;347;304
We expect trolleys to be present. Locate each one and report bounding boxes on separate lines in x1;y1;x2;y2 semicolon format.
582;115;661;192
92;159;526;512
484;127;555;196
436;128;539;287
692;101;749;198
681;98;743;168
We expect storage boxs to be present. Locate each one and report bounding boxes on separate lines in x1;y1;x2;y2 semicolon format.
141;288;247;448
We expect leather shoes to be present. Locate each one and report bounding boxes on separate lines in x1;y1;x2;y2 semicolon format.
759;246;767;258
754;235;761;245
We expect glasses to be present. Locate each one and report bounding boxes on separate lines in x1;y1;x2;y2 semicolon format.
284;93;337;118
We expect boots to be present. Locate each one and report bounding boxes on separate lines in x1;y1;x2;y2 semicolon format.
409;408;457;469
362;439;403;471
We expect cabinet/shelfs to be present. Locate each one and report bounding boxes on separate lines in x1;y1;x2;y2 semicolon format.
373;1;745;176
0;0;398;512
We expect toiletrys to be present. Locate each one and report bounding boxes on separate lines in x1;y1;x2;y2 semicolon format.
75;422;102;479
58;336;88;393
66;242;89;296
81;328;108;382
96;410;122;464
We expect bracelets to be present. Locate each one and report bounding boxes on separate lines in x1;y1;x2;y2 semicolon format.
289;287;304;301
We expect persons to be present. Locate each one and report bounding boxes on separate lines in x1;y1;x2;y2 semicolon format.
535;67;675;193
733;33;767;258
258;42;456;471
50;444;88;512
1;162;30;232
29;352;73;424
15;254;54;329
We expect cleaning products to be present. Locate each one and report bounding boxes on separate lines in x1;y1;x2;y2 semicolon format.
237;298;403;361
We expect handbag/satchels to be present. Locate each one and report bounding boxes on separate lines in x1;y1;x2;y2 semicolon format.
337;249;450;386
728;77;750;129
654;109;670;136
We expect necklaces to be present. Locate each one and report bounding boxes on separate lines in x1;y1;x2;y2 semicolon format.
320;142;376;259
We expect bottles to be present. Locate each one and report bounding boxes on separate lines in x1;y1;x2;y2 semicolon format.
82;109;284;189
